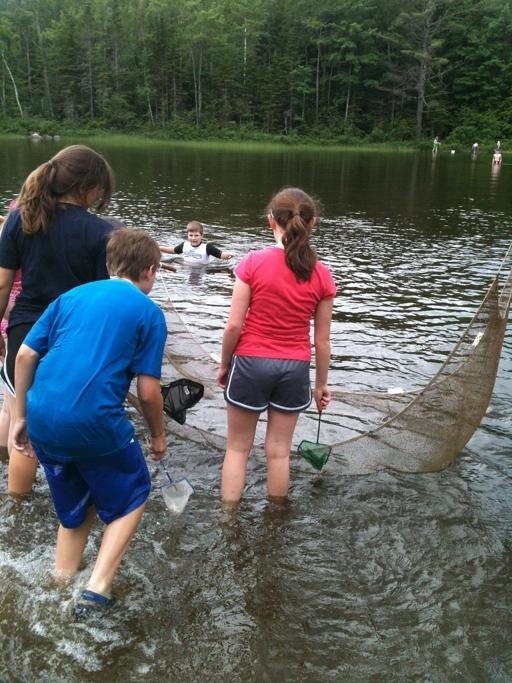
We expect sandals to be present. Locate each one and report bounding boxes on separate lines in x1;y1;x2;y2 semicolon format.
71;589;114;622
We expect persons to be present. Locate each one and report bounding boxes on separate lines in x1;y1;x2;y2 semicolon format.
151;429;164;438
157;219;236;266
0;199;25;449
214;183;339;507
11;226;169;628
0;141;126;500
432;135;440;152
471;142;478;154
491;148;502;165
